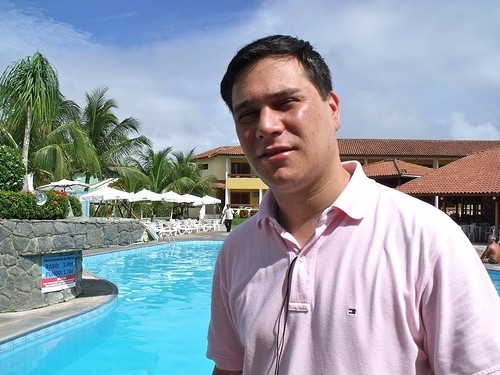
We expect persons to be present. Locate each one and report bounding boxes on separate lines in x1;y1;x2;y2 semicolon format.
222;204;235;231
205;35;500;375
480;235;500;264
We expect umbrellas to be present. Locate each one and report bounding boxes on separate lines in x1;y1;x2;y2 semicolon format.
201;193;221;218
179;193;206;219
79;186;134;216
36;178;91;193
127;188;175;219
160;190;194;219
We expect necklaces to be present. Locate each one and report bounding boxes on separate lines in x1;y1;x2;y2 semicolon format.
274;206;315;375
